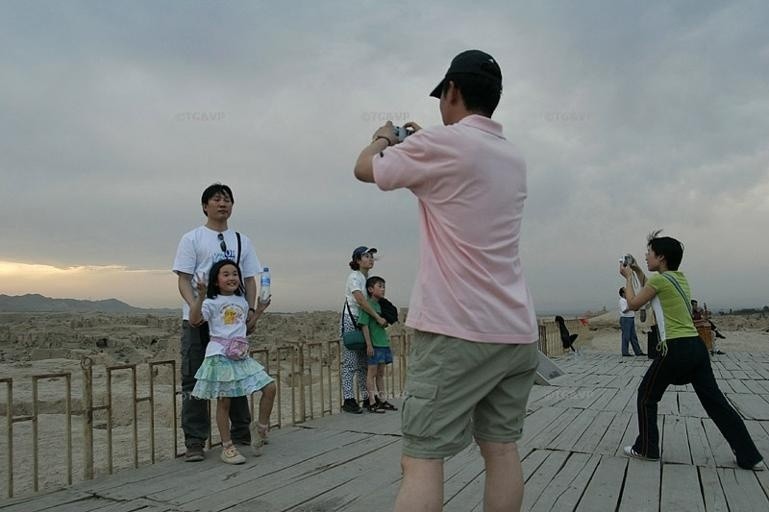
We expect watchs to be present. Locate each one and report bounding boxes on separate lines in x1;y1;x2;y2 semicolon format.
372;136;391;146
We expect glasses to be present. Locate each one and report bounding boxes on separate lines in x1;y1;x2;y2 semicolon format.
218;230;228;253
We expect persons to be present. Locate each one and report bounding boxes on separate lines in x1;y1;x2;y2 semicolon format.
188;270;276;465
357;276;398;414
341;247;388;413
173;185;269;462
356;50;535;512
691;300;726;355
621;237;766;470
619;287;648;356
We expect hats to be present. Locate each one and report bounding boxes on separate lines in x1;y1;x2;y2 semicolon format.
352;244;377;259
430;49;504;101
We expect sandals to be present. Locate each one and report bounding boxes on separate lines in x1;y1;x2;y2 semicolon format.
379;401;398;411
367;403;385;413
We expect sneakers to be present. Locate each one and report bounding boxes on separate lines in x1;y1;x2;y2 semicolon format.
220;446;247;465
342;397;363;414
363;395;380;408
249;421;269;458
622;445;658;462
232;433;269;446
183;443;205;462
748;460;765;471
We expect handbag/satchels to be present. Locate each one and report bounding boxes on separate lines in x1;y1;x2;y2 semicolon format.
342;300;367;351
648;273;698;385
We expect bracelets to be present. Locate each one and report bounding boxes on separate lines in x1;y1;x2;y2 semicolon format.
249;308;255;313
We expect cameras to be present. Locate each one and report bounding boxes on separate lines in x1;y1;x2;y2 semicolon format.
394;126;411;139
619;254;634;265
